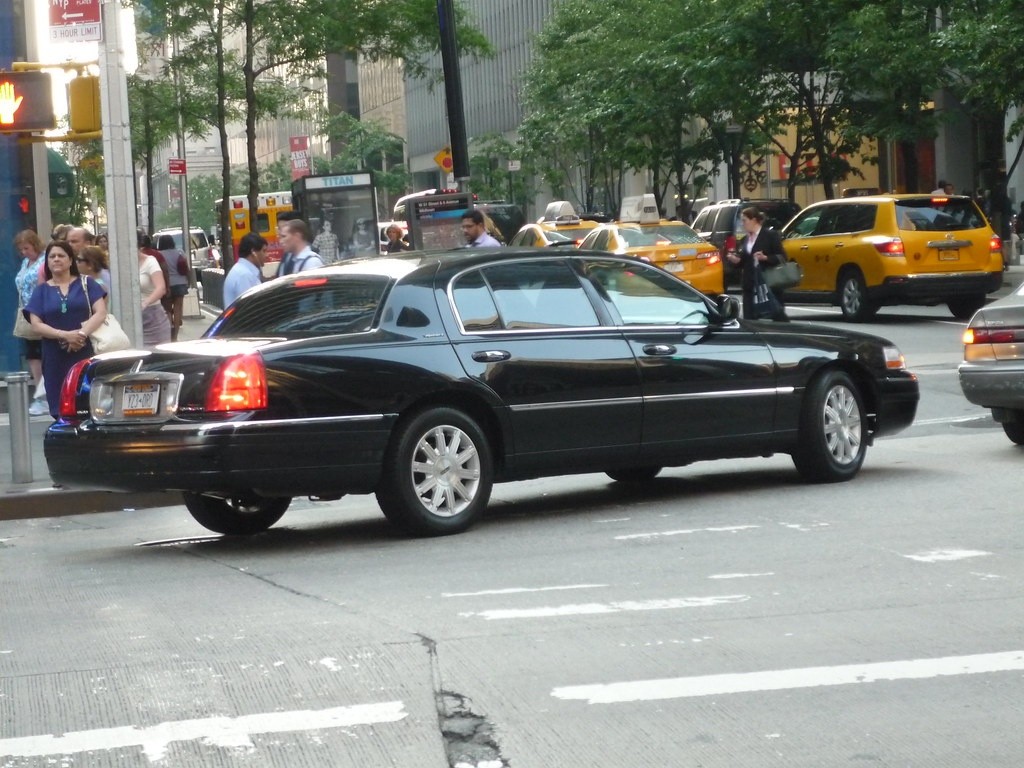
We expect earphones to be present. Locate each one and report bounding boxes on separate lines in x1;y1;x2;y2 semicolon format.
253;251;257;257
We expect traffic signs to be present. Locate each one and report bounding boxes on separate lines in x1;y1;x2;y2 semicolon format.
49;0;103;43
169;159;186;175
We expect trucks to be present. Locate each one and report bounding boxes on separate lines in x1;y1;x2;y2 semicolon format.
214;191;293;263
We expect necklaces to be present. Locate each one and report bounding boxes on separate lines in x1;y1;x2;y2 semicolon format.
53;278;71;314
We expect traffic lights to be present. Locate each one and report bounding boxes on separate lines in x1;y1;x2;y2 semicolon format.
0;69;56;133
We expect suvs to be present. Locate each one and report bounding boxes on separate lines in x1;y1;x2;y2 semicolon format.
475;200;526;247
152;226;217;283
691;198;803;294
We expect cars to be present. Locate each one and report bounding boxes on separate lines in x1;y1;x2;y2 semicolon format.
578;195;725;300
780;193;1005;320
507;202;600;250
958;281;1024;444
43;246;921;537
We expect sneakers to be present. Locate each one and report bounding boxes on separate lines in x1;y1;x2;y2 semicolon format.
29;401;50;417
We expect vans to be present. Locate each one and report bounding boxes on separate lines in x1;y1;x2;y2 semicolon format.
393;189;478;252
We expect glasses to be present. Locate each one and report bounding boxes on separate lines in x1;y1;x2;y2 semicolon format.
76;256;88;264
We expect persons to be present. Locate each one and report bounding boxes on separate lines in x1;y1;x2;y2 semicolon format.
311;218;375;263
461;210;501;247
137;227;190;346
276;211;324;277
931;180;990;217
727;208;789;321
223;232;269;309
386;225;408;253
15;223;111;418
669;201;697;226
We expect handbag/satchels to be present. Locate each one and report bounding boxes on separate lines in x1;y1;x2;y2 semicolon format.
13;308;44;340
177;256;187;276
743;262;782;319
761;253;802;290
81;275;131;354
